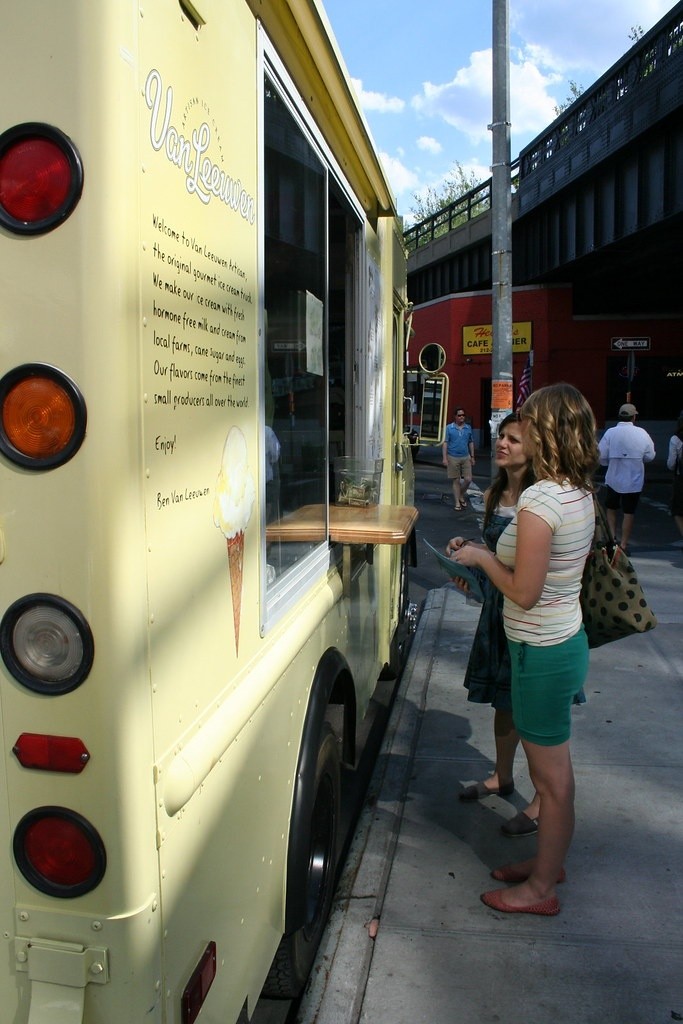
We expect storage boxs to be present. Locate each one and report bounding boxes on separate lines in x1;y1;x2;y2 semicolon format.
334;455;384;507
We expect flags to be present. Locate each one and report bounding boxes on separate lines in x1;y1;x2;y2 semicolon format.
516;351;531;413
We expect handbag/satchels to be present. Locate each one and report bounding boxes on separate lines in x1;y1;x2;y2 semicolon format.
579;477;658;650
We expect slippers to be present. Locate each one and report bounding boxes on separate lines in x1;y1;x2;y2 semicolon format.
459;499;468;510
454;504;462;511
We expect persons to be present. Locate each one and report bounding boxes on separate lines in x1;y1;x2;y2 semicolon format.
450;384;596;916
667;410;683;535
443;407;474;510
596;404;656;557
265;423;281;525
446;412;541;836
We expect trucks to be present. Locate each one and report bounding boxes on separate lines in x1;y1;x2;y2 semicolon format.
0;0;448;1024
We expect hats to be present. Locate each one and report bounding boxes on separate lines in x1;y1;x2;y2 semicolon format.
618;403;638;416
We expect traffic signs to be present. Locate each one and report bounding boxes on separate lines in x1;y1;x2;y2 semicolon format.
611;336;651;350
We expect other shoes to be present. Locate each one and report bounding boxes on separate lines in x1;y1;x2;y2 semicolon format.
480;889;561;915
500;811;539;837
619;544;632;557
458;779;514;801
491;863;567;883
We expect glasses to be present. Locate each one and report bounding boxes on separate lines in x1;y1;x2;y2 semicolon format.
457;414;466;417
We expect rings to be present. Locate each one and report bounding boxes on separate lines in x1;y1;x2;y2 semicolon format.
456;557;460;562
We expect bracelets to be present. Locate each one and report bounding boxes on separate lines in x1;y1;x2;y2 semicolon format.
471;456;474;458
